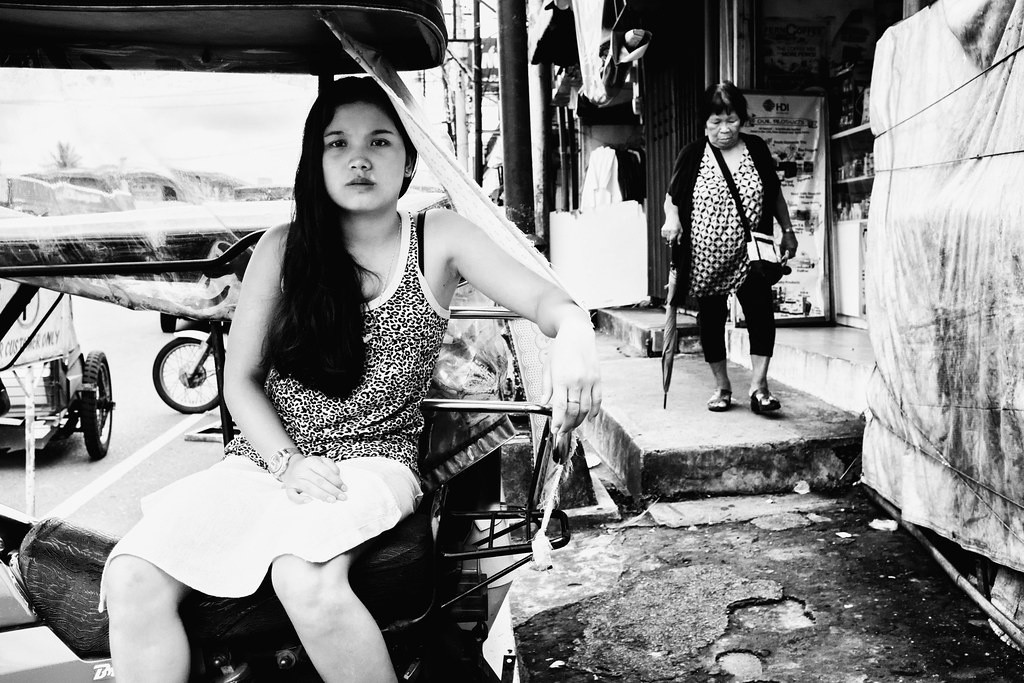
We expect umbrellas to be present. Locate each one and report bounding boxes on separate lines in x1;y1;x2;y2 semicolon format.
659;267;678;409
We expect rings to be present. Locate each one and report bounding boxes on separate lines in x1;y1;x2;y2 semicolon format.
567;399;580;404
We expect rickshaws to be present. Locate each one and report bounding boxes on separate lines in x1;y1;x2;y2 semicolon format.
0;0;621;683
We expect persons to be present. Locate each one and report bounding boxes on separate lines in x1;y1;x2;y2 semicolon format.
661;80;798;413
97;76;602;683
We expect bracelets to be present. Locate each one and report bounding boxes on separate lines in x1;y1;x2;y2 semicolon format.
783;228;793;233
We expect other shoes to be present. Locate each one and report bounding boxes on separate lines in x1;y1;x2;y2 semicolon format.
708;389;732;412
751;388;781;413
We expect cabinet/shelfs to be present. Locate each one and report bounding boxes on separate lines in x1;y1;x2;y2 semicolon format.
829;63;875;184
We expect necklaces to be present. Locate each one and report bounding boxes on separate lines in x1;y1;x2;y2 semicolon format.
363;219;401;343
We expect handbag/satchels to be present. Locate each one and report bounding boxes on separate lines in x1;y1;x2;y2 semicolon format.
745;230;782;283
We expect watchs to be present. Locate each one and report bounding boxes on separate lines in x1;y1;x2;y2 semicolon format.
268;448;301;479
